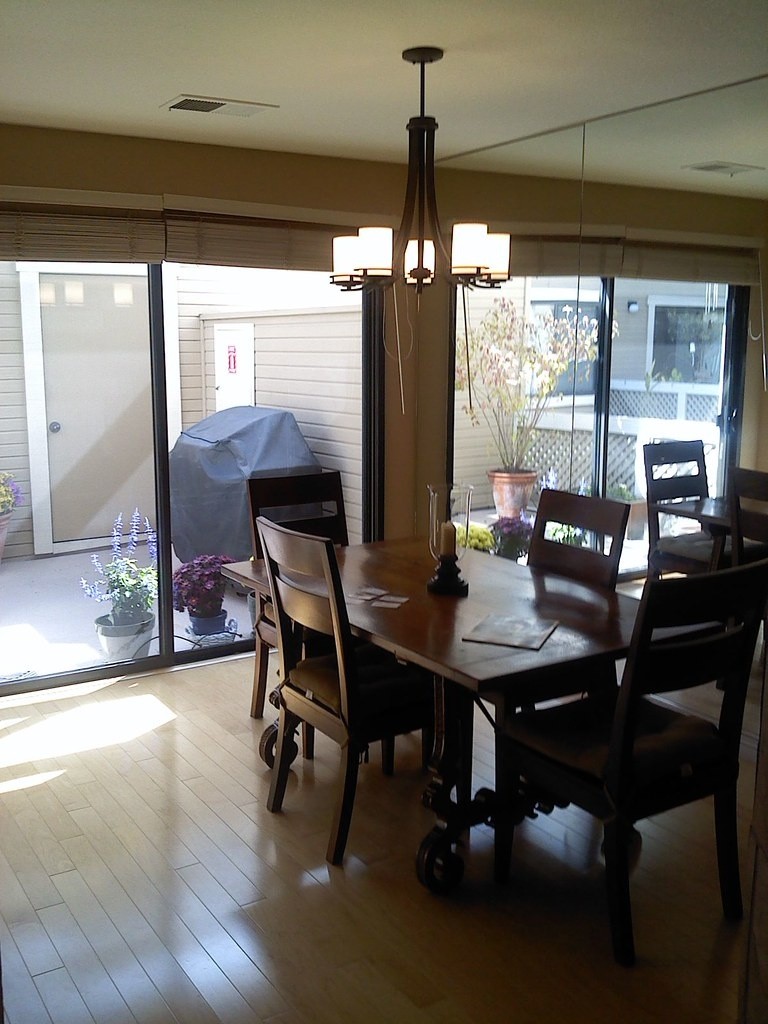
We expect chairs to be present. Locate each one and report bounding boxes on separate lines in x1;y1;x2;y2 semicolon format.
490;557;767;972
256;514;472;867
726;467;768;567
246;471;349;762
642;440;757;578
527;488;630;590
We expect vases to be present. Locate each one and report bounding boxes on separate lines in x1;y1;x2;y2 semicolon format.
0;511;14;565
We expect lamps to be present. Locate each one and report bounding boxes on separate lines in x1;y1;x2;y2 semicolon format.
422;476;471;595
331;47;511;296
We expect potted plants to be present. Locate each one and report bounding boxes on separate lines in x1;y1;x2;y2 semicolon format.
93;613;156;660
188;609;229;636
458;297;620;518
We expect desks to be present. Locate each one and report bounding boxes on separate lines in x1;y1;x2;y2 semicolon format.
221;530;725;886
651;495;768;569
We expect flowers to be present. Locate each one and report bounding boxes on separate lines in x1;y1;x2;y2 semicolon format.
0;473;24;516
174;554;234;618
80;507;158;626
487;516;533;553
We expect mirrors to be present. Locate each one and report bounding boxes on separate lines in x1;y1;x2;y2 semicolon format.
414;74;767;744
